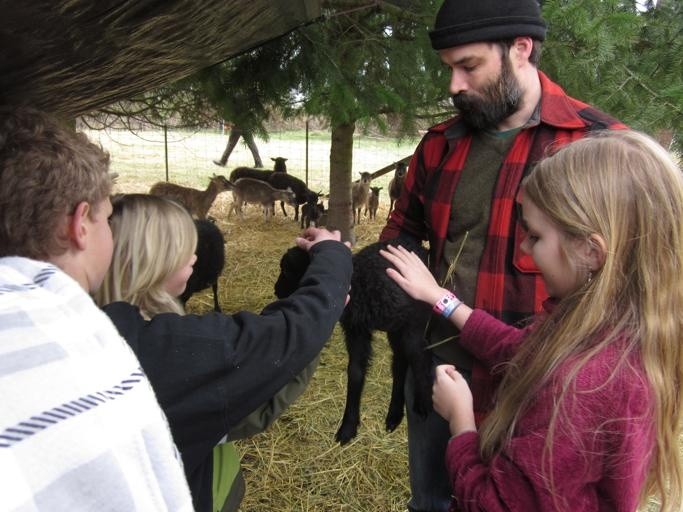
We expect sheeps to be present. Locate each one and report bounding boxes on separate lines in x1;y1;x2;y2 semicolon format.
177;216;226;313
274;239;434;447
149;172;235;221
228;157;325;229
364;186;383;221
386;162;409;223
351;172;377;225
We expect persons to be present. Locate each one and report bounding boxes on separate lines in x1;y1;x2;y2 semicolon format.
0;106;197;511
384;128;682;511
378;1;637;511
211;285;354;512
212;118;264;168
94;194;354;511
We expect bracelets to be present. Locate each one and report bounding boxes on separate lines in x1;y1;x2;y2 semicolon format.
433;290;455;314
440;298;462;319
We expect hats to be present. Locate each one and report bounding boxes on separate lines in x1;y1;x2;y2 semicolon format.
429;1;547;51
